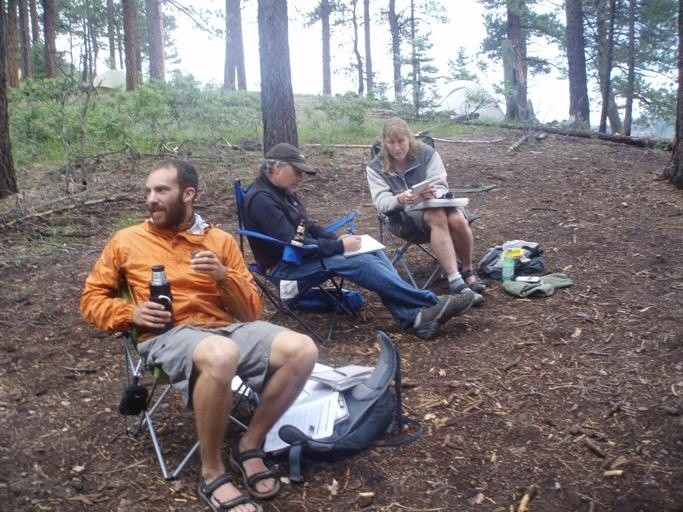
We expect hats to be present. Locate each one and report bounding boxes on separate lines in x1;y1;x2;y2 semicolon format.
264;143;316;176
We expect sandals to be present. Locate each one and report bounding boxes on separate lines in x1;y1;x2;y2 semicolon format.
449;284;485;307
228;441;281;500
196;472;264;512
461;269;485;292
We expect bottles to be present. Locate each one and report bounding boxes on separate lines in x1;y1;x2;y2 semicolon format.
290;218;307;246
501;248;516;281
149;265;173;335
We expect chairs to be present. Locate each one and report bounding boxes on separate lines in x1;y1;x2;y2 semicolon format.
371;136;497;293
233;179;361;348
108;278;259;480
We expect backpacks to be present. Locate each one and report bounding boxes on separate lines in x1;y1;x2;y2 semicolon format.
478;239;545;278
271;332;424;484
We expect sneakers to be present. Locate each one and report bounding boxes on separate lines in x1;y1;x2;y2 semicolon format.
412;294;454;341
450;287;474;317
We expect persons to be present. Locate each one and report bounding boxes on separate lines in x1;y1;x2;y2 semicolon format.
79;160;319;512
243;143;474;341
366;118;486;305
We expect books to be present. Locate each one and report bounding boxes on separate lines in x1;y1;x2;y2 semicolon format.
411;179;430;194
343;233;386;257
413;197;468;209
